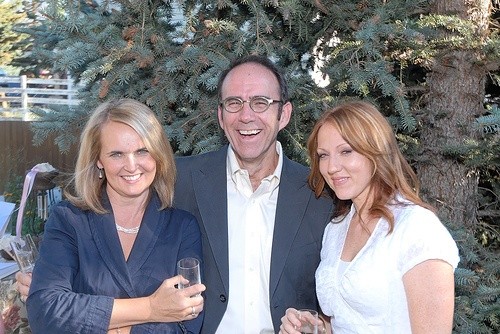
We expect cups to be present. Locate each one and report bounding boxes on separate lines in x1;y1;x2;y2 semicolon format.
177;258;201;298
296;309;318;334
10;234;39;273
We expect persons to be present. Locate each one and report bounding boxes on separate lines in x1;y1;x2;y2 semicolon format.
26;98;206;334
278;100;460;334
15;55;337;334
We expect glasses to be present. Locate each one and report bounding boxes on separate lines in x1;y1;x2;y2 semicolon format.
219;96;284;113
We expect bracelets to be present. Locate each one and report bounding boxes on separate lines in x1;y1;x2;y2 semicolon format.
318;316;327;334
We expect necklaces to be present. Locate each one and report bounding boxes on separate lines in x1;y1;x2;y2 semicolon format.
115;224;140;235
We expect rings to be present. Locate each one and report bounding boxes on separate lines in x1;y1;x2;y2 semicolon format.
192;307;197;318
